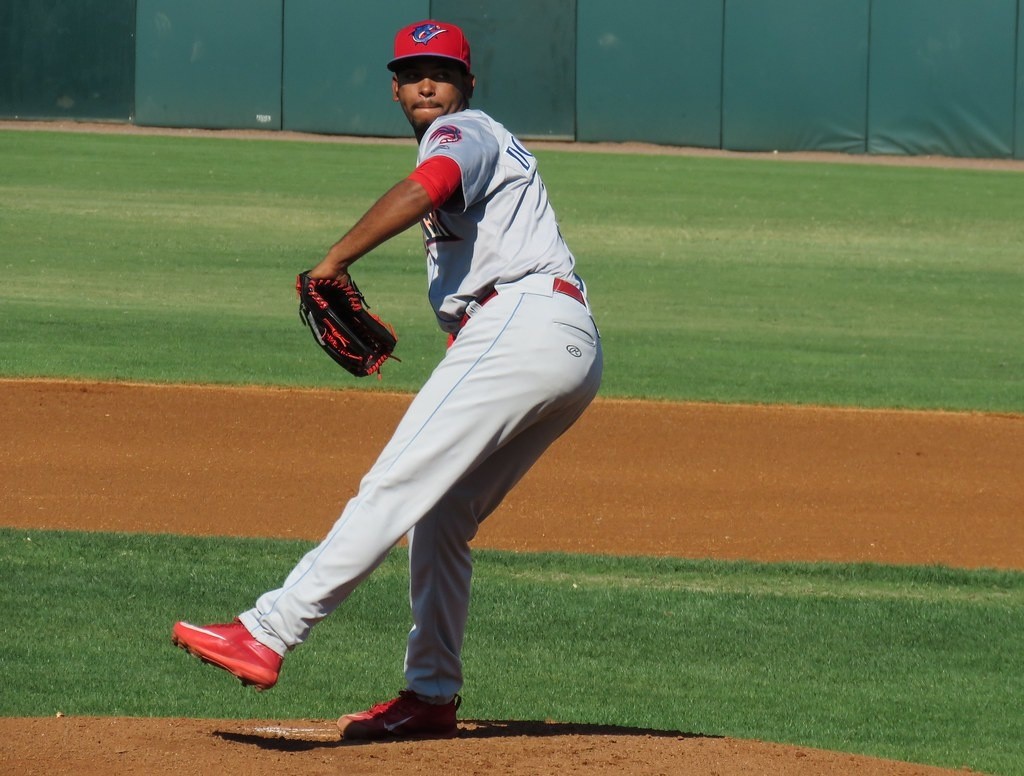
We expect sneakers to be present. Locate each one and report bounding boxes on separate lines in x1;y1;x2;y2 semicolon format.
172;618;284;693
337;690;463;742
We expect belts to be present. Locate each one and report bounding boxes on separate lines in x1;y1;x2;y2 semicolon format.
459;277;586;329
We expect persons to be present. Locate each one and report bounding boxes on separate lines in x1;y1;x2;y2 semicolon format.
172;20;604;739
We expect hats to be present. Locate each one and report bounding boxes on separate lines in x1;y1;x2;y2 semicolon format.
386;20;471;81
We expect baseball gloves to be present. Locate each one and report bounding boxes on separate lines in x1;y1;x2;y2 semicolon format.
294;270;395;377
